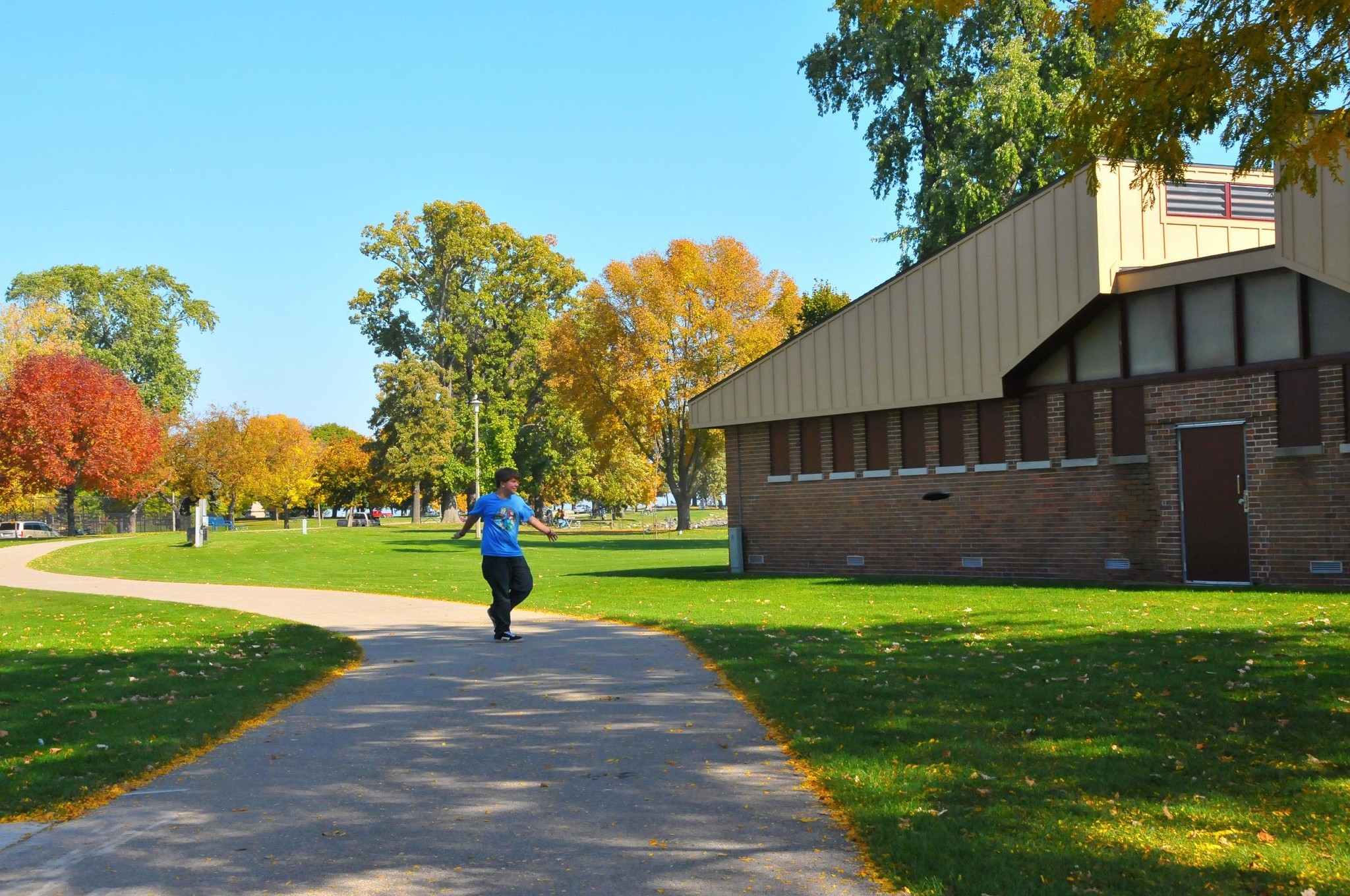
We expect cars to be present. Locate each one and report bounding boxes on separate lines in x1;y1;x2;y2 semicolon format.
574;505;591;514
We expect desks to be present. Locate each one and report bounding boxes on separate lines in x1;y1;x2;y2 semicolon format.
563;517;576;529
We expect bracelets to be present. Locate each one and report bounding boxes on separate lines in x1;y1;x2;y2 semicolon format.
547;528;551;535
459;532;465;536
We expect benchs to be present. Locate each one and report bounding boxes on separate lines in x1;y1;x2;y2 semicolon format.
208;516;233;532
558;521;563;523
575;521;581;523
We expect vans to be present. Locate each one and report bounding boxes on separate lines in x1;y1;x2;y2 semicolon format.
0;521;56;538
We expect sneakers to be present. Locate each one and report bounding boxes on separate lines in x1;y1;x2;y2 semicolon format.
494;630;524;643
487;608;496;629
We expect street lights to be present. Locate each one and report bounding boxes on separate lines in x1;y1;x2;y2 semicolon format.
471;400;483;538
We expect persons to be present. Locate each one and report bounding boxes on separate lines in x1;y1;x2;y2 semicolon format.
554;510;566;528
546;509;554;526
450;467;558;642
561;510;565;519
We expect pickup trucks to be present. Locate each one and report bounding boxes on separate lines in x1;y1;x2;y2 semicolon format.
372;510;393;518
337;512;380;527
